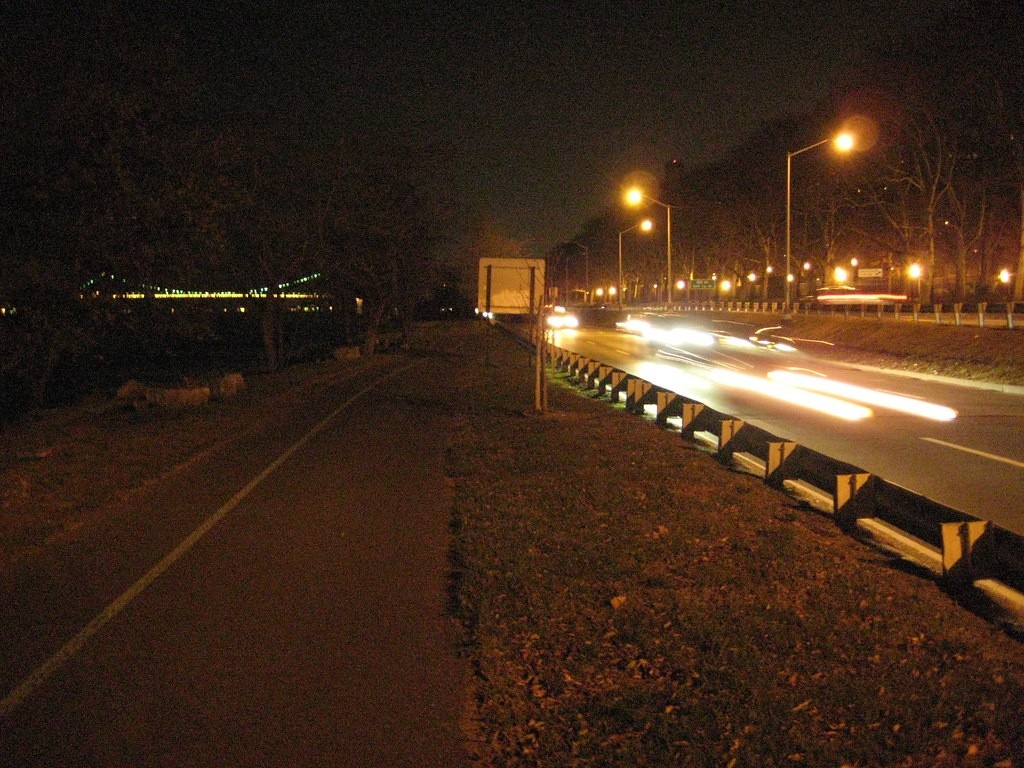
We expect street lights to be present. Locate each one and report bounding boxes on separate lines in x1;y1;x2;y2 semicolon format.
625;186;672;305
560;239;591;293
615;219;651;307
783;133;854;312
